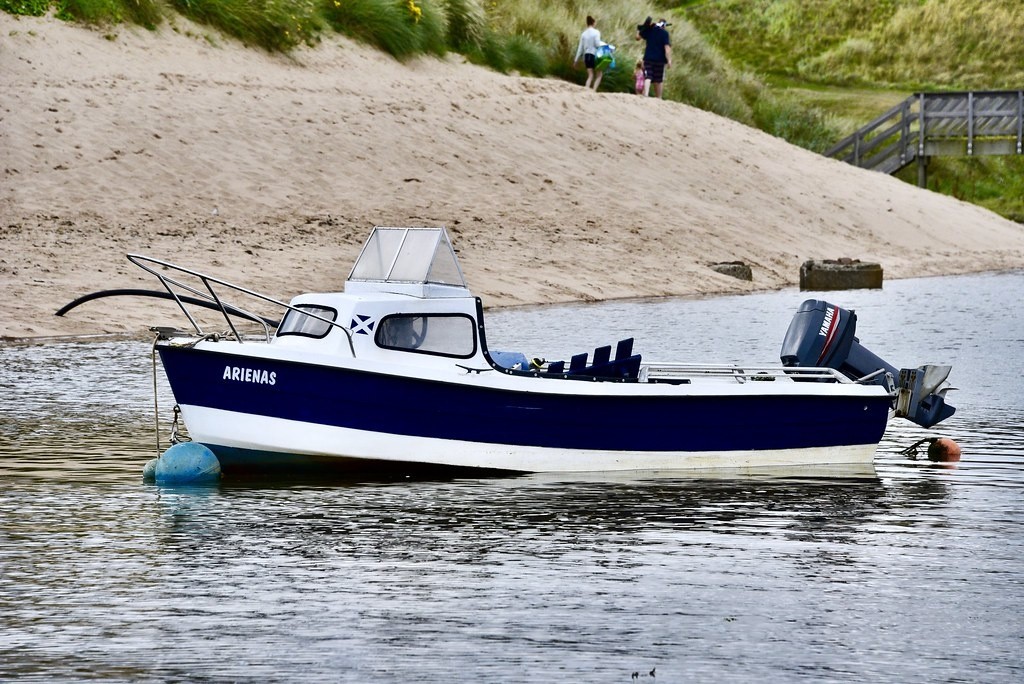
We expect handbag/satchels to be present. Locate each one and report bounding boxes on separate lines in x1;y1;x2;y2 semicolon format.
593;45;616;74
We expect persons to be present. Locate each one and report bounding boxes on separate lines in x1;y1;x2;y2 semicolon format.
636;19;672;99
574;16;614;90
632;61;644;95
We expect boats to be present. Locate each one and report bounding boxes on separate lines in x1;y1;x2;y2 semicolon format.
119;226;962;483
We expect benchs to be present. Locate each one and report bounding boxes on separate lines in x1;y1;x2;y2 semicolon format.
547;338;642;382
